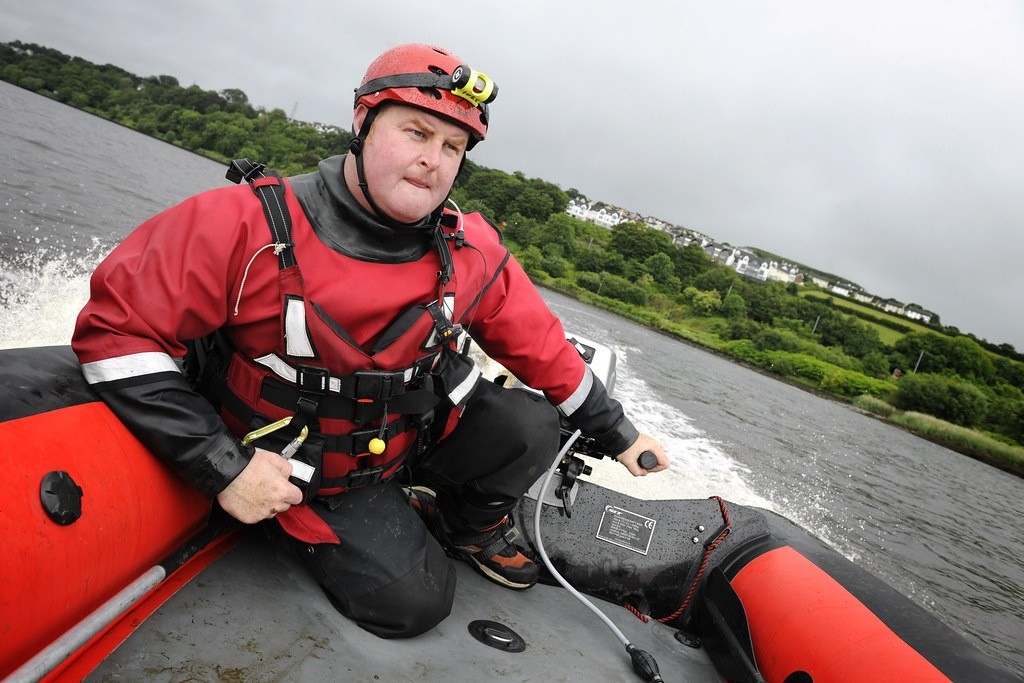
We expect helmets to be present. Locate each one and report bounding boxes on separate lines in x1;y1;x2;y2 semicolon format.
355;45;489;151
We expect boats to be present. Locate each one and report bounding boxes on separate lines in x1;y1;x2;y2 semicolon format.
0;330;1024;683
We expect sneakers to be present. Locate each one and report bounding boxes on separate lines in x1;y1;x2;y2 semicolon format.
432;503;537;589
397;484;452;529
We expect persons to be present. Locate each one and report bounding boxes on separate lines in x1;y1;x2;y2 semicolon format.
71;44;670;639
